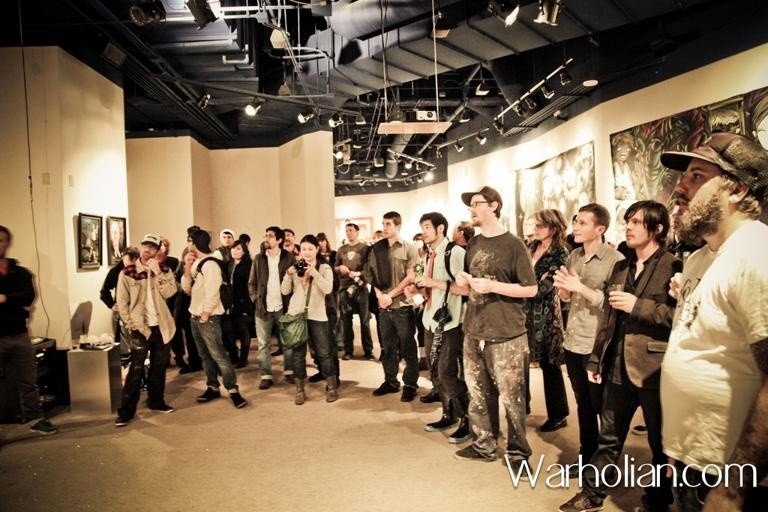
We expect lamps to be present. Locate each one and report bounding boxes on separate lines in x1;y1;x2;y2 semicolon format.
103;0;599;193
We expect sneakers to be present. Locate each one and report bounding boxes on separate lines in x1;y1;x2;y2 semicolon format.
401;385;417;401
163;354;249;375
259;350;341;404
147;405;174;414
364;353;376;361
31;418;60;435
537;416;674;511
371;381;398;396
229;392;248;408
195;387;222;402
114;413;135;427
342;353;352;361
419;387;533;478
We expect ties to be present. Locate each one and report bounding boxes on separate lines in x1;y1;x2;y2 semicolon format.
425;251;437;311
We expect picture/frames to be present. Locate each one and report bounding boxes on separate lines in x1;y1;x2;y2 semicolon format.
79;213;102;270
336;216;373;246
107;216;126;265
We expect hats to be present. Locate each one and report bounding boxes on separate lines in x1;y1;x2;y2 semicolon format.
660;133;768;200
121;229;237;257
462;186;502;213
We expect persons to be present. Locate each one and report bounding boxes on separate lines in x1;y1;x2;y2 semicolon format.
100;132;768;512
0;225;58;435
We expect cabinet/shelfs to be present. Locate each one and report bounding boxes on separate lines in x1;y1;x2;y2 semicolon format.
67;343;123;415
13;337;55;424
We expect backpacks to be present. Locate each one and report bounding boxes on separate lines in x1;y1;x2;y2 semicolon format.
197;258;233;311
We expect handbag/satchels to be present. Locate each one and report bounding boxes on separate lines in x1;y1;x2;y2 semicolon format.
279;309;309;349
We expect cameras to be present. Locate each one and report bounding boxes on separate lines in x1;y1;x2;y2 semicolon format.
294;258;307;277
